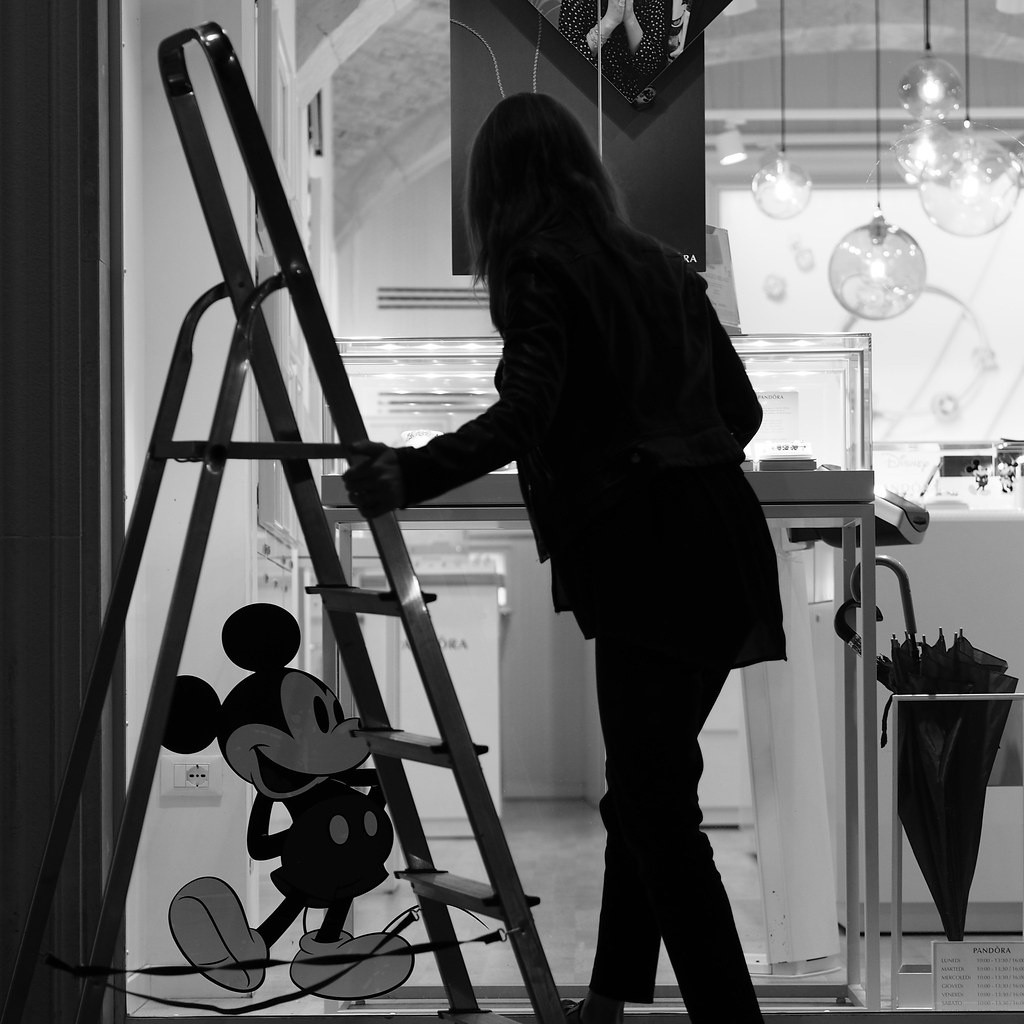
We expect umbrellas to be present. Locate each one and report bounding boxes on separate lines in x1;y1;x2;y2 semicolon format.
834;555;1018;941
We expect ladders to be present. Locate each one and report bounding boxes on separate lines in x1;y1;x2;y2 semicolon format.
1;18;569;1024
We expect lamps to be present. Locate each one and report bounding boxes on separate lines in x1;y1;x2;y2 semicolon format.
751;0;1024;323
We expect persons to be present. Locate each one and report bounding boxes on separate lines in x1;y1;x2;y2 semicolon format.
558;0;673;103
339;92;789;1021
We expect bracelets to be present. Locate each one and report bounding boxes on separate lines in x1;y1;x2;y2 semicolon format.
588;24;611;49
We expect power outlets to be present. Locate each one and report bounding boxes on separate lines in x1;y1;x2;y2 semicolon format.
157;757;231;801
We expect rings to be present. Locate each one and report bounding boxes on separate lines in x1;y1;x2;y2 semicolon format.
618;2;625;7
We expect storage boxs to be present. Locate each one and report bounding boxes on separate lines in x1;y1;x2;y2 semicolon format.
887;688;1024;1012
